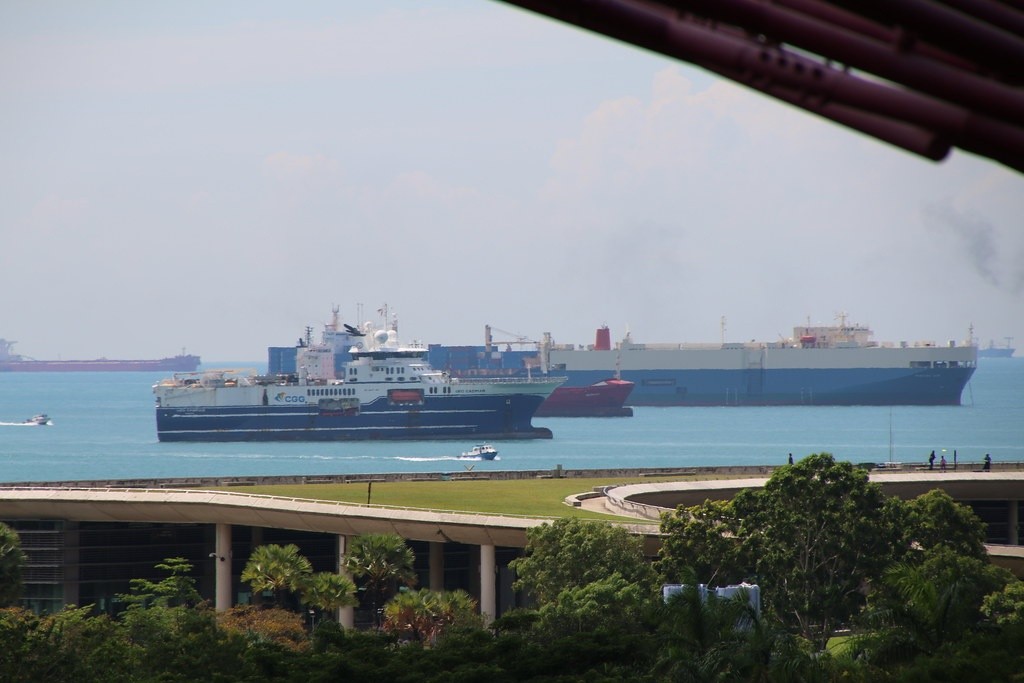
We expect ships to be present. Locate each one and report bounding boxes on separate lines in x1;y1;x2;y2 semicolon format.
424;310;980;406
0;339;202;372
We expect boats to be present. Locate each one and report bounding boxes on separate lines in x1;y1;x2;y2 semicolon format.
532;377;635;417
389;389;422;405
321;408;359;418
23;414;51;424
978;336;1016;359
152;301;554;442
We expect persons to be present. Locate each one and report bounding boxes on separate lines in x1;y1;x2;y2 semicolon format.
983;454;992;472
928;450;936;470
940;456;947;473
788;452;794;465
